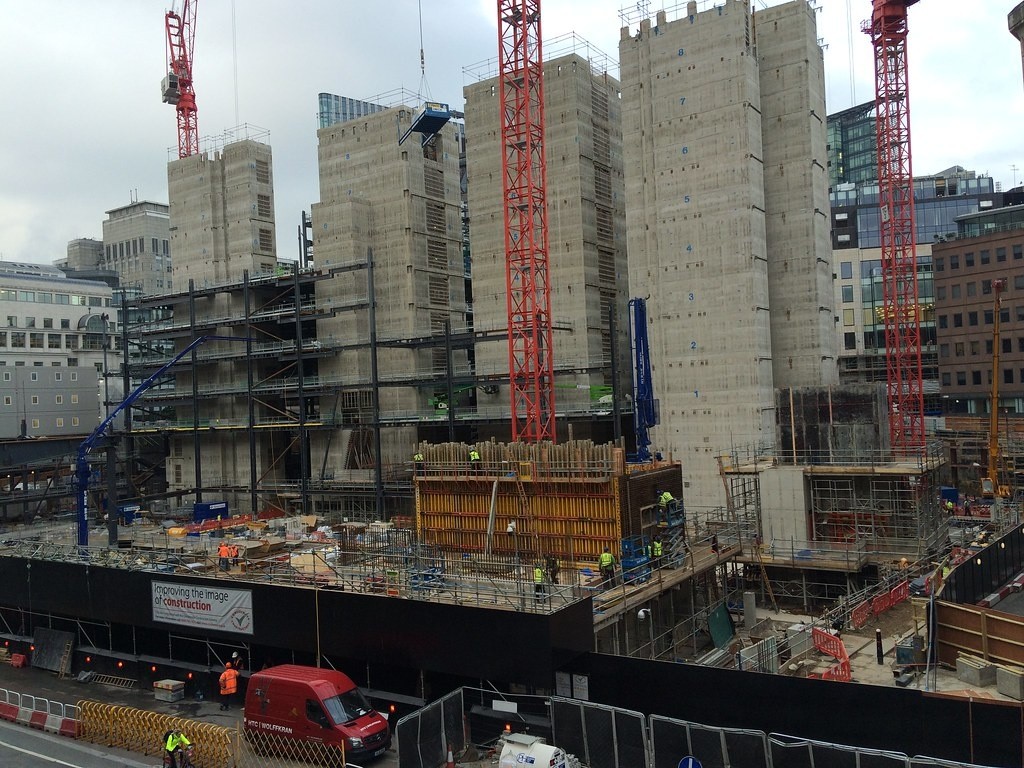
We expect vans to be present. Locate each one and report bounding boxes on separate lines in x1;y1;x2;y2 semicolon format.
243;665;393;767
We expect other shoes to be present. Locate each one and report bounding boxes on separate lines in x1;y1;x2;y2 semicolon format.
225;707;229;711
220;705;224;710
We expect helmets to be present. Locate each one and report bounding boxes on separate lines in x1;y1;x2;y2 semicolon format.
226;662;232;667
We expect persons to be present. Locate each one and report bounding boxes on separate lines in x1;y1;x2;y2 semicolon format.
414;452;423;477
964;500;971;515
544;554;559;584
642;536;662;578
534;563;546;603
598;547;617;590
232;652;243;669
468;447;481;476
218;513;222;520
947;498;954;515
166;730;190;768
218;662;239;709
217;542;239;571
655;489;679;523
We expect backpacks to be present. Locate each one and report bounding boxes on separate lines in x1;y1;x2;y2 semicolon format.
163;730;175;743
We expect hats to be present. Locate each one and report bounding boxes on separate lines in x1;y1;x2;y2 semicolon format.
232;651;238;658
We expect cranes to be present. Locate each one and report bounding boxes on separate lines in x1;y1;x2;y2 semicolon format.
861;0;923;457
160;0;206;160
495;2;558;444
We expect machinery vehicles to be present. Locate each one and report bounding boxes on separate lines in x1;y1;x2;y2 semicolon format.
980;276;1014;503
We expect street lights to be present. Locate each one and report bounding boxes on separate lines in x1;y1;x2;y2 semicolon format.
637;609;655;660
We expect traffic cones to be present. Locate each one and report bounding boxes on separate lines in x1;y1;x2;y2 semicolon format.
444;744;456;768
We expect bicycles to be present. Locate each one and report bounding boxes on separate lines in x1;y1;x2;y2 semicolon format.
163;746;195;768
